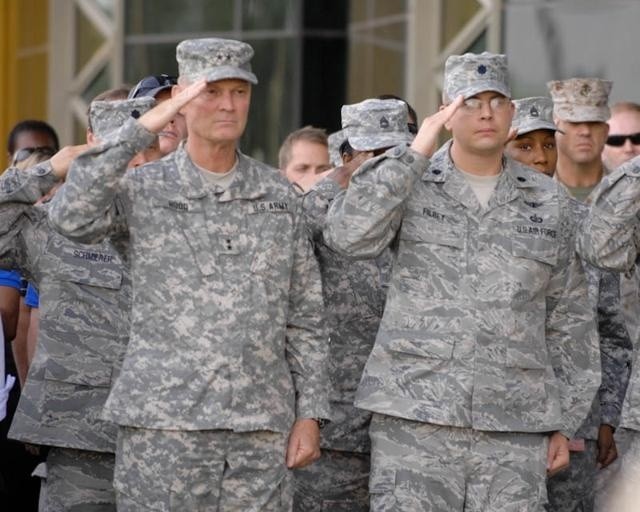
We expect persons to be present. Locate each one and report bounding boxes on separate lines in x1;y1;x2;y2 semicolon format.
128;73;184;154
51;36;324;507
600;103;640;171
279;126;329;188
303;98;415;506
379;93;420;137
506;97;558;176
0;265;40;510
0;96;160;501
576;154;640;273
5;120;57;151
324;53;600;507
549;77;636;512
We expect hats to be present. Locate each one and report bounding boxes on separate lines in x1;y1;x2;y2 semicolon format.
441;50;513;106
508;94;567;137
326;131;347;165
86;94;156;144
547;73;615;124
175;38;259;87
338;97;415;152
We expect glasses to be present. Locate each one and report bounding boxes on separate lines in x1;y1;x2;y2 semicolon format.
607;134;640;145
460;97;509;116
408;121;418;135
14;146;55;163
129;74;177;100
